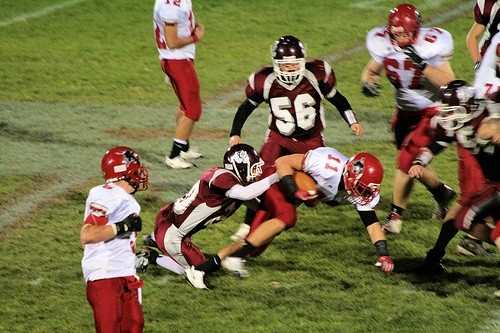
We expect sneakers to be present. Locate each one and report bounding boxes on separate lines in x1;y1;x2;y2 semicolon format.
434;186;454;218
179;148;205;158
164;156;194;169
185;266;211;291
222;256;251;276
136;234;157;272
386;212;401;235
458;236;494;258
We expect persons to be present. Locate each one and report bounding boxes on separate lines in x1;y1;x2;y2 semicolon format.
362;3;465;235
80;145;151;332
152;0;205;170
217;144;398;274
134;142;284;281
405;77;500;281
228;34;364;236
465;1;499;68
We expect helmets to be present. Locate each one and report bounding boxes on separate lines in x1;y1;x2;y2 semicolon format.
389;4;421;41
444;79;475;113
101;146;143;187
274;35;308;60
344;152;384;195
224;144;261;183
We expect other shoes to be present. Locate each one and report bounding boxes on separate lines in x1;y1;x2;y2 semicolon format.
419;258;456;280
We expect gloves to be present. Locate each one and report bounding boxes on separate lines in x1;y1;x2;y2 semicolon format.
359;82;385;97
294;189;318;200
403;47;425;69
376;256;396;273
113;212;142;235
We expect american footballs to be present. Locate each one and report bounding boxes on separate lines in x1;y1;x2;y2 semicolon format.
291;172;317;196
